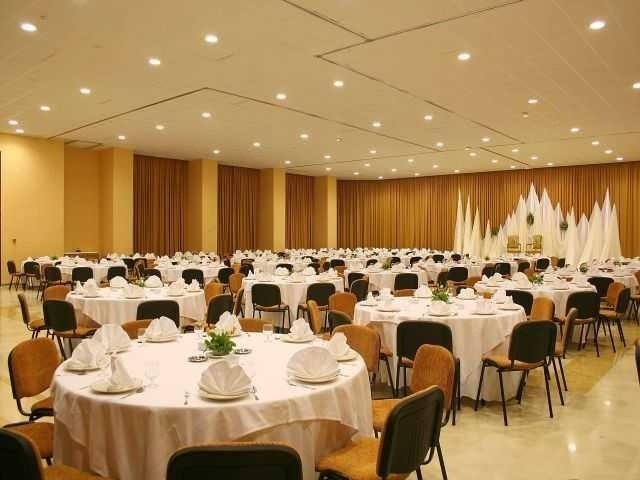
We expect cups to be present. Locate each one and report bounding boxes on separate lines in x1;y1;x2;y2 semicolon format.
262;320;273;336
192;320;205;334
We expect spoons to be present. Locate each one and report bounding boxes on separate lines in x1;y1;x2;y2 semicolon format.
183;392;189;405
250;386;259;401
120;387;143;400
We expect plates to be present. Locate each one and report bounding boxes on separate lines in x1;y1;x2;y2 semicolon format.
65;365;100;373
331;352;359;362
104;346;126;354
92;377;143;393
359;295;521;317
167;263;225;269
293;375;337;384
198;388;248;400
70;285;202;299
144;337;177;343
352;268;422;276
560;268;632;278
478;279;591;290
243;272;340;283
281;335;316;342
226;332;242;337
68;265;74;267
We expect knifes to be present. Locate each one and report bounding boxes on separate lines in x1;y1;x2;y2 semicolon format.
287;382;315;389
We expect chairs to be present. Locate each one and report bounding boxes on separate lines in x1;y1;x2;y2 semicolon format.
0;247;639;480
